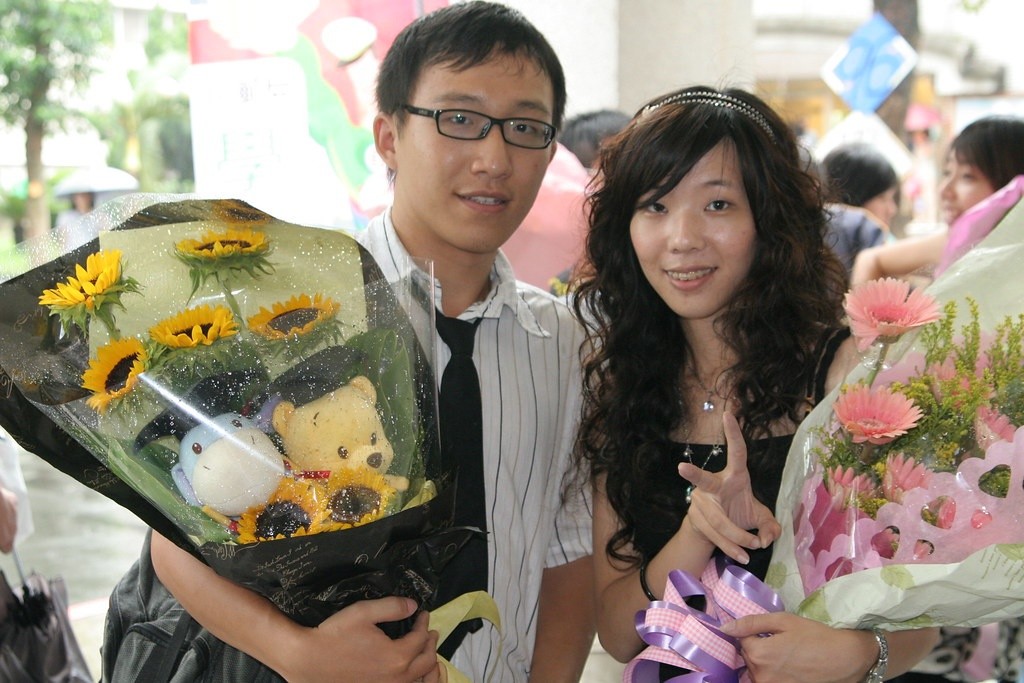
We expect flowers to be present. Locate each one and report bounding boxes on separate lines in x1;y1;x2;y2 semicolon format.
34;227;345;420
793;276;1023;585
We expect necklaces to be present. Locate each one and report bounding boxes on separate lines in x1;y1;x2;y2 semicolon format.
686;365;731;411
674;387;725;503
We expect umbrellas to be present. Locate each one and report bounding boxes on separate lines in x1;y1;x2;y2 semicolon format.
1;549;95;683
54;165;139;197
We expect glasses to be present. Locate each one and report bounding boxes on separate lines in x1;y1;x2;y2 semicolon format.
398;102;557;149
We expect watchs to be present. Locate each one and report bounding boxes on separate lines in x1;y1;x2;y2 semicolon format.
867;630;889;683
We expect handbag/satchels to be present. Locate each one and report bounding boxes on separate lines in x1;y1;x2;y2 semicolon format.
98;523;284;683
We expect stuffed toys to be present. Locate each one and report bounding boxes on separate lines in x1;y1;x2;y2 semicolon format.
133;346;409;530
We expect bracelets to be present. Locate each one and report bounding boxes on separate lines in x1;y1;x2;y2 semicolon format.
639;562;657;602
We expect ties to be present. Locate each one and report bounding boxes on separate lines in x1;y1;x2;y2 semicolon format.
403;275;489;635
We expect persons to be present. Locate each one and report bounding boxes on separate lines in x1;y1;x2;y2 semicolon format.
559;88;945;683
822;143;899;226
801;161;891;301
559;110;630;169
848;114;1024;290
56;193;100;251
149;0;596;683
0;424;35;555
